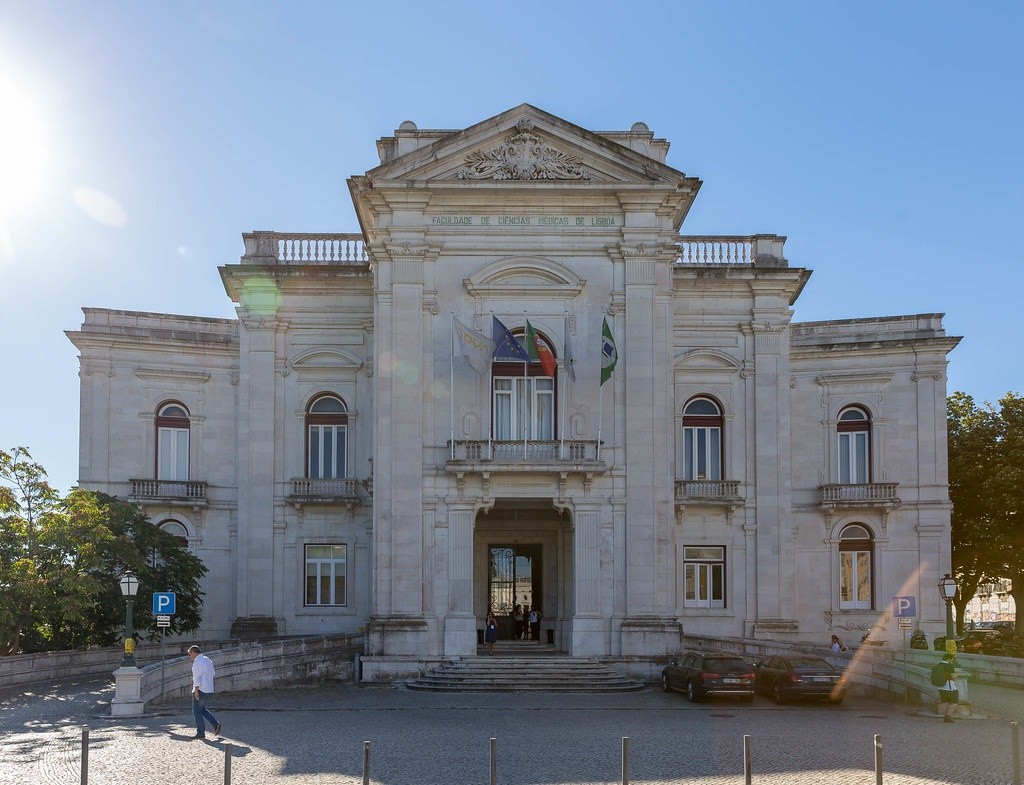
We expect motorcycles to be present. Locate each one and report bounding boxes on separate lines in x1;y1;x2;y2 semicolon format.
909;629;929;650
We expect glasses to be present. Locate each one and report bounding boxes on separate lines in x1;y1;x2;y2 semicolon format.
189;652;192;656
488;613;492;615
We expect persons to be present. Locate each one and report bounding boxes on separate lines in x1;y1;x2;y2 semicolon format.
187;645;222;739
970;619;976;630
513;604;543;641
831;635;845;653
936;653;961;723
485;612;498;656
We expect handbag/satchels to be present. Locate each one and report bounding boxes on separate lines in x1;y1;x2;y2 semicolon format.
836;640;850;653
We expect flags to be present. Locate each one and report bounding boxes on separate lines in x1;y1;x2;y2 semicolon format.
493;314;532;363
452;316;497;376
564;315;576;382
527;317;557;377
600;316;618;386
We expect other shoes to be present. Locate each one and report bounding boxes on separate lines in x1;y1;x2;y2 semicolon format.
215;722;221;735
944;715;956;723
190;735;205;740
488;651;495;655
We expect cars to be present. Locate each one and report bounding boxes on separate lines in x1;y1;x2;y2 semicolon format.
753;654;848;706
660;650;756;703
963;620;1016;639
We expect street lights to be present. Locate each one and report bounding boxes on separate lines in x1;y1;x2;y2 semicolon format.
119;569;139;668
936;573;962;669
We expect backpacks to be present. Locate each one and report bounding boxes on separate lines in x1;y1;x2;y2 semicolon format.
931;663;949;686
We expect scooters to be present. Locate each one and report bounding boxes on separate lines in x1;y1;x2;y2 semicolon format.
933;635;1024;658
860;628;887;646
